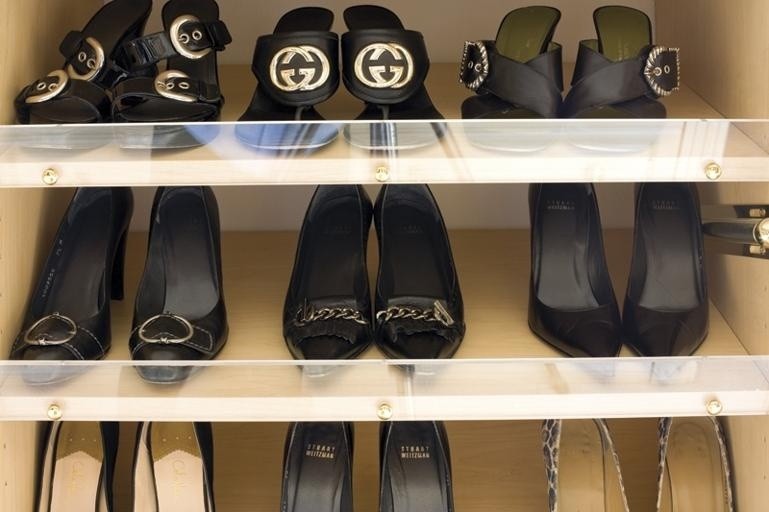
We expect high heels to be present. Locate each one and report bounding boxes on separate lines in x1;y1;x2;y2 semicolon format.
9;188;134;387
129;186;230;386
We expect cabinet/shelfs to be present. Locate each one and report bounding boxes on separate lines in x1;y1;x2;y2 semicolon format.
1;2;769;512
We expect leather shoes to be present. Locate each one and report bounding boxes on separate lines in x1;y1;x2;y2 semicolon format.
621;182;710;383
527;184;621;383
540;419;630;512
131;422;216;511
378;422;455;512
373;185;467;380
278;422;356;512
34;422;118;512
656;417;736;512
282;185;375;379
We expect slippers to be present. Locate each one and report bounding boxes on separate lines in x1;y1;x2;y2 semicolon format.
111;0;232;150
343;4;449;154
14;0;152;152
234;6;341;155
460;6;563;155
566;5;682;157
